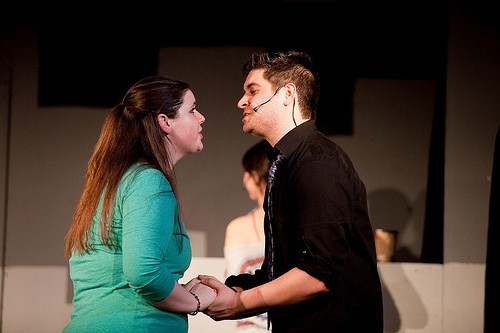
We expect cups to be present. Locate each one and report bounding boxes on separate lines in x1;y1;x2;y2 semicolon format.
374;229;398;262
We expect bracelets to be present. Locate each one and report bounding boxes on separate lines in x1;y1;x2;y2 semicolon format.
186;291;201;316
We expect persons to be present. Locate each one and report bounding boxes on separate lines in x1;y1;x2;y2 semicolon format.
60;75;218;333
222;139;273;333
197;50;384;333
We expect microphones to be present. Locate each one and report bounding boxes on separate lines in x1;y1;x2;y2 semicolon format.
254;100;270;112
